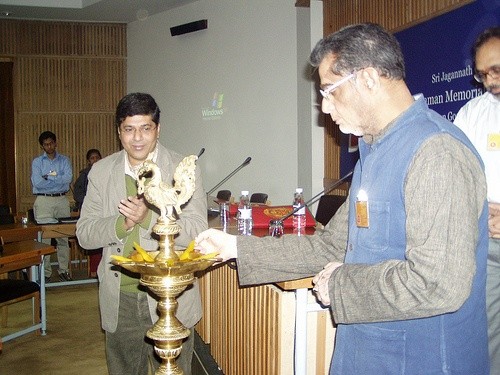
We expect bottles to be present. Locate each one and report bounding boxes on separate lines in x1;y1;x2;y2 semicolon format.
237;190;253;236
292;188;306;234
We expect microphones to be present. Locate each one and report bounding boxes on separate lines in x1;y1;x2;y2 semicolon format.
206;157;251;194
198;148;205;159
279;168;356;221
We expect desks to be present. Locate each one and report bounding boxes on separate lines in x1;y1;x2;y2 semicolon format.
13;222;102;279
0;240;55;342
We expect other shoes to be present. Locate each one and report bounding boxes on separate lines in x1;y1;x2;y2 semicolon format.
60;272;72;281
45;277;50;281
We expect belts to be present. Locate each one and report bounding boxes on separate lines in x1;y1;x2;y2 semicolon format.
33;193;65;197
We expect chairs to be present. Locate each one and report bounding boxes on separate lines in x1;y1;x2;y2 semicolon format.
315;195;346;226
0;272;41;352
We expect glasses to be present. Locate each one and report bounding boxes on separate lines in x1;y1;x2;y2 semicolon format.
319;70;363;99
43;142;54;146
119;126;157;135
473;67;500;83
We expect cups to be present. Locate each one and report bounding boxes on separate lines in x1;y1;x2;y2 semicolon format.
268;219;284;238
219;203;231;223
21;217;29;224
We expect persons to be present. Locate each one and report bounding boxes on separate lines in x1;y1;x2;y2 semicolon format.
193;23;491;375
74;91;208;375
450;24;500;375
31;131;73;283
73;149;102;214
